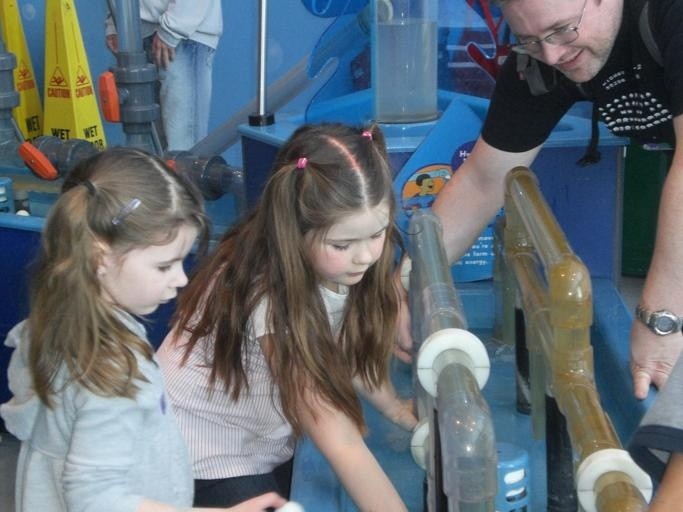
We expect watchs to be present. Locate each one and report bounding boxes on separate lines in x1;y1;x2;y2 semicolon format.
634;305;682;337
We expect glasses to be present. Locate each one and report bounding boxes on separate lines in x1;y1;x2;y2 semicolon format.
509;1;587;56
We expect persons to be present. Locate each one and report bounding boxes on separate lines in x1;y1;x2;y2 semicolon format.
154;120;420;512
1;146;289;512
390;0;683;400
626;348;683;512
102;0;223;152
